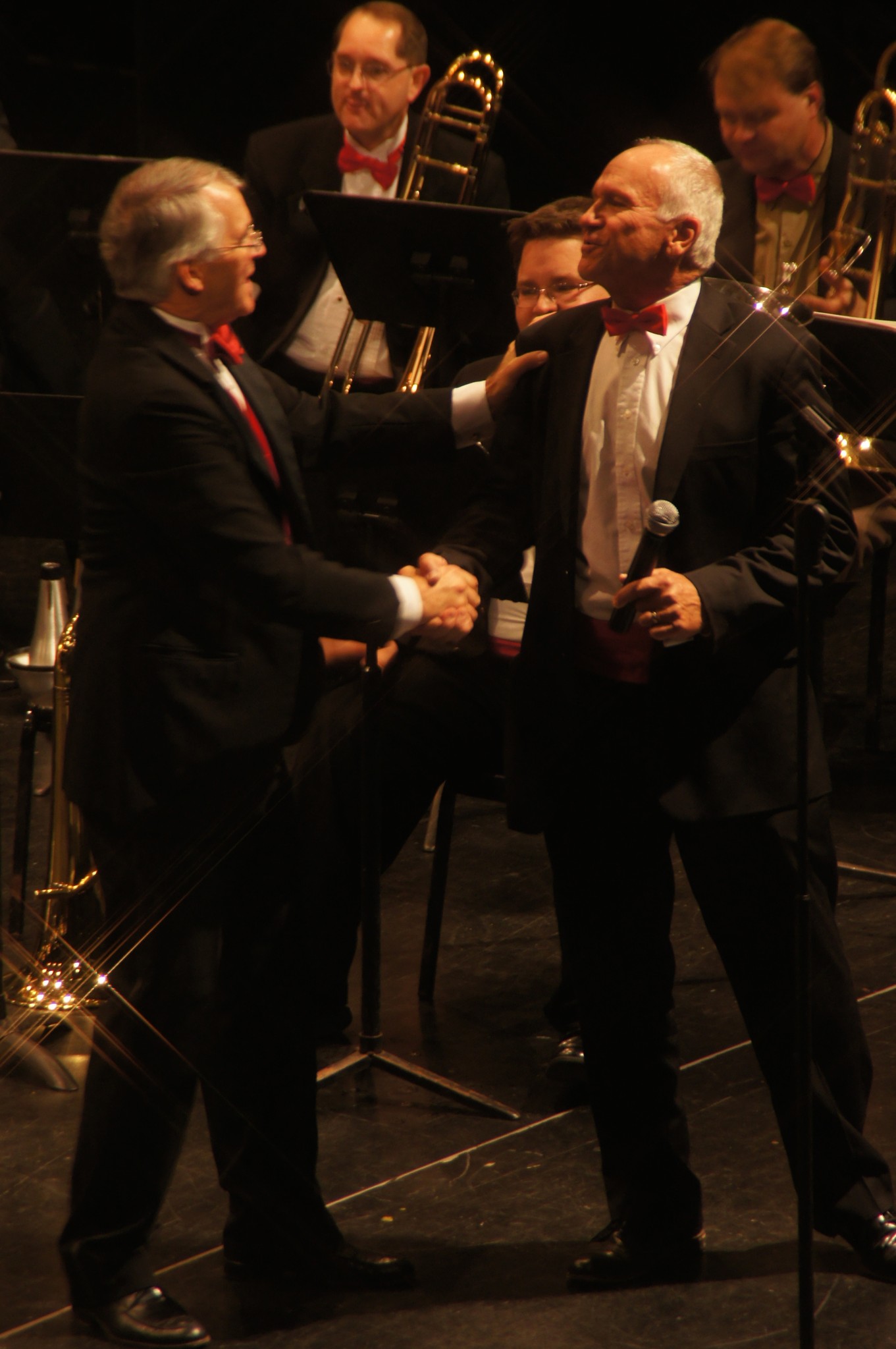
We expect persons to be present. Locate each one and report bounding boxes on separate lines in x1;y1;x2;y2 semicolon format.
703;17;896;320
269;194;610;1046
397;136;896;1292
235;0;517;396
52;156;552;1347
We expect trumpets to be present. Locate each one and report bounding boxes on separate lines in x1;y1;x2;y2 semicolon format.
6;611;98;1009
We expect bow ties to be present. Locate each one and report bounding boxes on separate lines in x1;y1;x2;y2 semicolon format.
173;323;244;365
754;175;816;205
337;136;407;191
601;304;668;336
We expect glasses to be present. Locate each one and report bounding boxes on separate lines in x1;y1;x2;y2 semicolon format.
511;282;599;309
167;224;264;273
330;52;414;83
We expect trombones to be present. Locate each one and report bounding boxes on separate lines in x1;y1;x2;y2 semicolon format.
774;46;896;473
315;49;506;398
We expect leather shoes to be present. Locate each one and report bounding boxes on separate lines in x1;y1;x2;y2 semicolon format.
841;1208;896;1279
569;1227;707;1285
547;1035;585;1072
73;1283;212;1348
322;1251;418;1295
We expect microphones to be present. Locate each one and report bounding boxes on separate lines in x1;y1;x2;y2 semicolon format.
606;500;681;635
